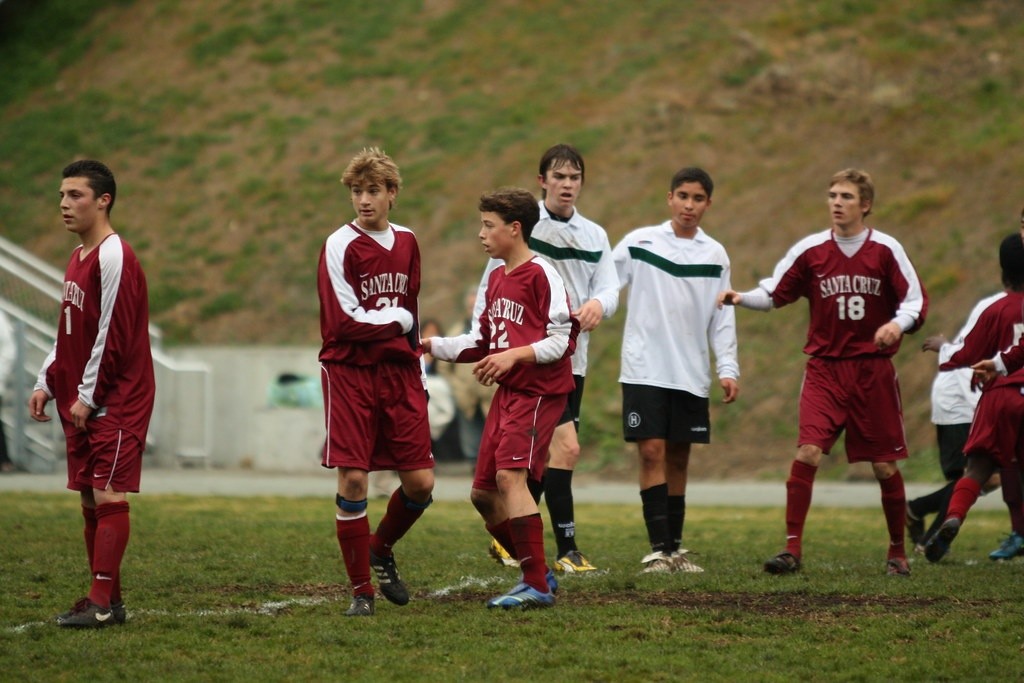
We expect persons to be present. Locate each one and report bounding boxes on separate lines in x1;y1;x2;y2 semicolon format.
0;308;16;474
30;160;155;628
612;167;741;574
417;283;498;476
316;147;435;618
716;169;929;574
488;143;620;572
906;207;1024;561
419;186;581;611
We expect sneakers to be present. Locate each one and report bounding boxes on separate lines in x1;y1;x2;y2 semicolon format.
54;597;125;628
344;593;375;615
487;568;558;610
368;535;411;604
989;531;1024;560
489;538;520;568
903;500;925;545
925;518;960;563
554;550;597;573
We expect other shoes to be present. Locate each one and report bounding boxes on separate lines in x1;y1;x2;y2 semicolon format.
641;552;673;572
887;559;911;577
765;552;799;574
671;549;705;572
